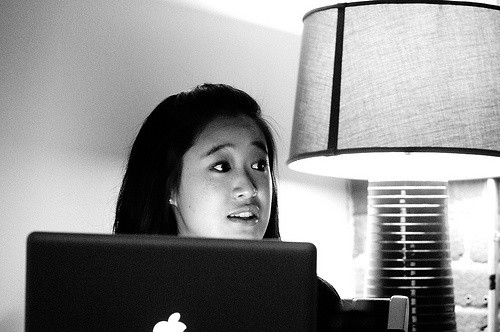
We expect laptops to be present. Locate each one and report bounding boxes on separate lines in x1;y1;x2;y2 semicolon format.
25;232;317;332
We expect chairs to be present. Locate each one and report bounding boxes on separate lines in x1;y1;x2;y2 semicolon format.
340;296;408;332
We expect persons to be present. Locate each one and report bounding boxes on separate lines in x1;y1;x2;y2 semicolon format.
112;82;343;331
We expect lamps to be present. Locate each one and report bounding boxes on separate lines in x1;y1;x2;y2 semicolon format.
285;1;499;331
